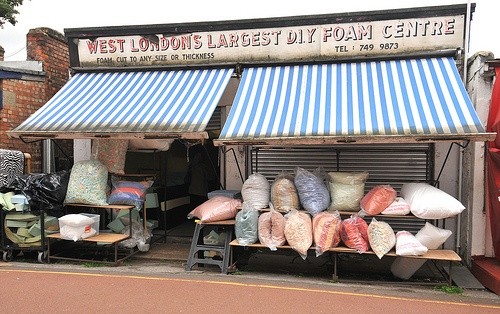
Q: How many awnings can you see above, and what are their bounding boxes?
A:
[11,67,234,139]
[212,57,497,147]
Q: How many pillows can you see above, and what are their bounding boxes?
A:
[187,167,467,260]
[109,177,154,210]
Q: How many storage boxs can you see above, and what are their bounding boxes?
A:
[58,213,100,240]
[3,192,30,211]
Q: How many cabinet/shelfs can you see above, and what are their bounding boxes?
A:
[47,199,147,264]
[228,206,462,287]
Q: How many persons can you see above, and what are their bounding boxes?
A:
[184,153,214,224]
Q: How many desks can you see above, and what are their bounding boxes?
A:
[187,220,235,274]
[0,209,48,251]
